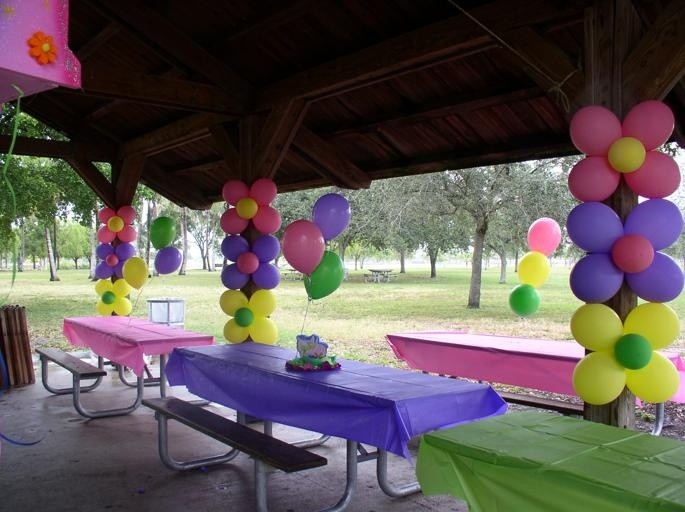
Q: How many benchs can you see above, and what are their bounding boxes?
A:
[142,393,326,512]
[494,389,588,417]
[281,266,398,283]
[34,346,103,421]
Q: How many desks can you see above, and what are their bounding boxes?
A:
[164,337,498,510]
[63,308,214,414]
[407,404,684,510]
[380,328,685,436]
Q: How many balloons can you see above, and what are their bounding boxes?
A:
[220,177,350,344]
[94,206,182,317]
